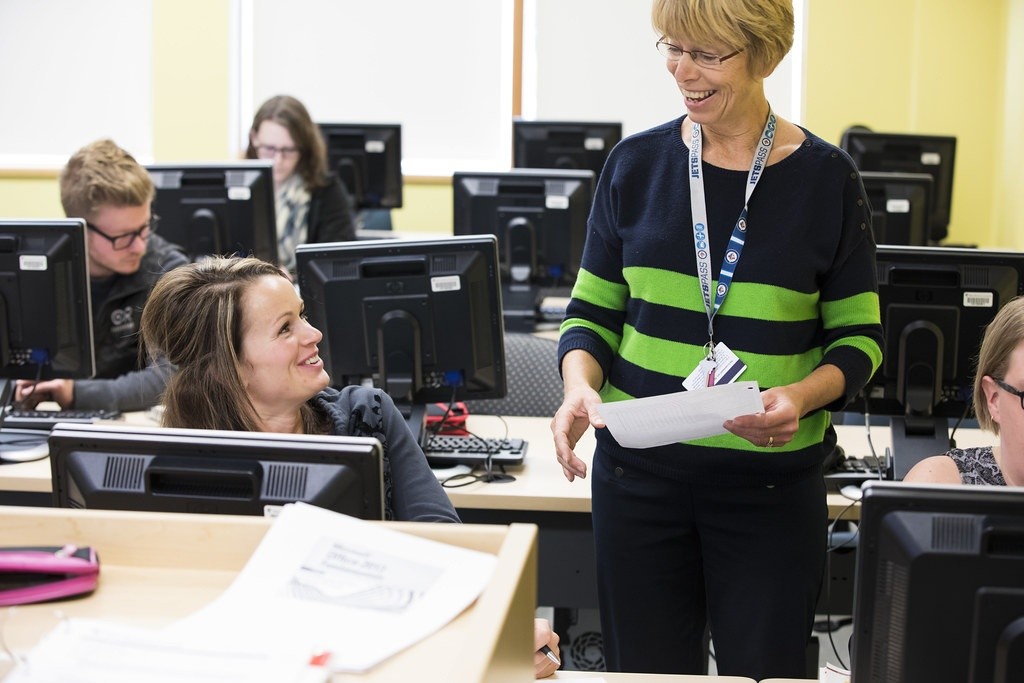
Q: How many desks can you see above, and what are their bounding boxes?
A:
[0,502,539,681]
[0,364,994,520]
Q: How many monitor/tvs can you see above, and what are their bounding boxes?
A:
[0,123,1024,683]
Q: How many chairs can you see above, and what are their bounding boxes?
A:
[451,331,566,418]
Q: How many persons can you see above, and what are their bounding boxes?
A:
[901,296,1024,490]
[243,92,359,281]
[19,137,193,414]
[139,251,560,678]
[554,1,884,680]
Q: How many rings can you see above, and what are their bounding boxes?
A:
[767,436,774,446]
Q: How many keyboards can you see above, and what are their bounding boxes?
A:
[825,454,893,489]
[425,434,529,466]
[3,408,121,429]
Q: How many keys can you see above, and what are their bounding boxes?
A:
[704,358,717,375]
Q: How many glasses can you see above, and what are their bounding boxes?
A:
[86,213,161,250]
[656,34,754,69]
[255,144,306,161]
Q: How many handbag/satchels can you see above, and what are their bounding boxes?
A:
[0,546,99,607]
